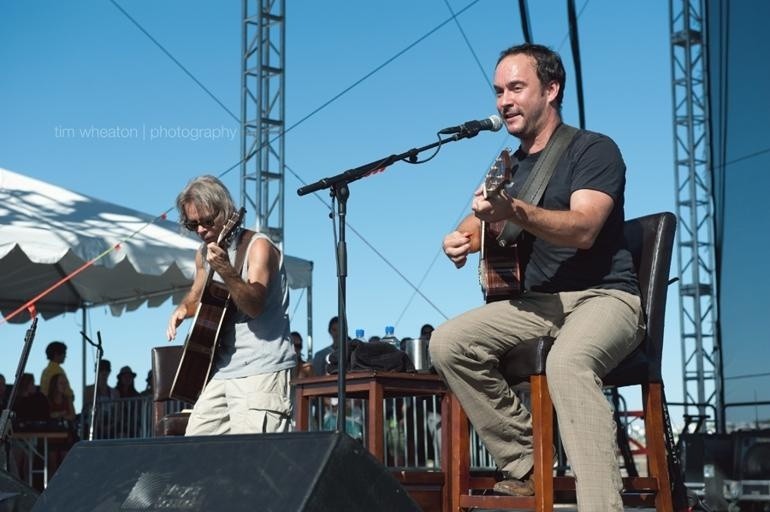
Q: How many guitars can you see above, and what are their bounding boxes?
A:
[168,208,246,404]
[476,148,520,302]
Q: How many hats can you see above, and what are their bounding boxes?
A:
[146,370,152,381]
[117,367,136,380]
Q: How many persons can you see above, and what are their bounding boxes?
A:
[141,367,154,439]
[83,359,121,441]
[166,173,300,439]
[114,365,143,438]
[15,373,49,420]
[40,340,76,420]
[0,375,11,418]
[290,314,440,467]
[10,373,38,484]
[427,41,646,512]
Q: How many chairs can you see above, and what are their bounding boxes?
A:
[450,211,678,511]
[150,344,192,436]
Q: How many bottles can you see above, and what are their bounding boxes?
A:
[379,326,401,352]
[353,329,368,343]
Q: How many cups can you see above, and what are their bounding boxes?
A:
[404,338,433,374]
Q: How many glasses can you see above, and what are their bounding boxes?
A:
[184,211,220,231]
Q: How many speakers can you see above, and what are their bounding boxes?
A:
[0,466,39,511]
[732,428,770,510]
[686,434,733,511]
[26,430,419,512]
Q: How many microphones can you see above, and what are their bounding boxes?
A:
[442,115,503,140]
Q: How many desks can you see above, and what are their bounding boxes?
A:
[9,432,71,489]
[287,368,451,512]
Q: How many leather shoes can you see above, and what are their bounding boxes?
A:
[490,443,562,498]
